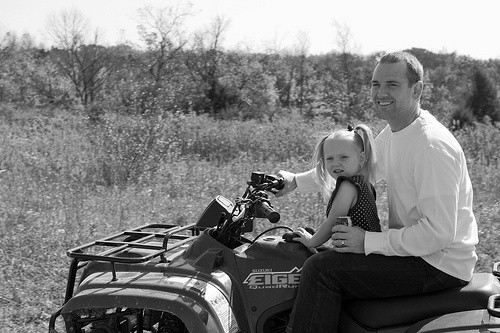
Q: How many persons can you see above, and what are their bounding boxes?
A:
[273,50,479,332]
[293,123,381,254]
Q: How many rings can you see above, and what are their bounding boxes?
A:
[343,238,345,246]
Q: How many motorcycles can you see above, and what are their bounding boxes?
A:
[48,170,500,333]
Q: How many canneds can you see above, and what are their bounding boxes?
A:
[334,217,352,247]
